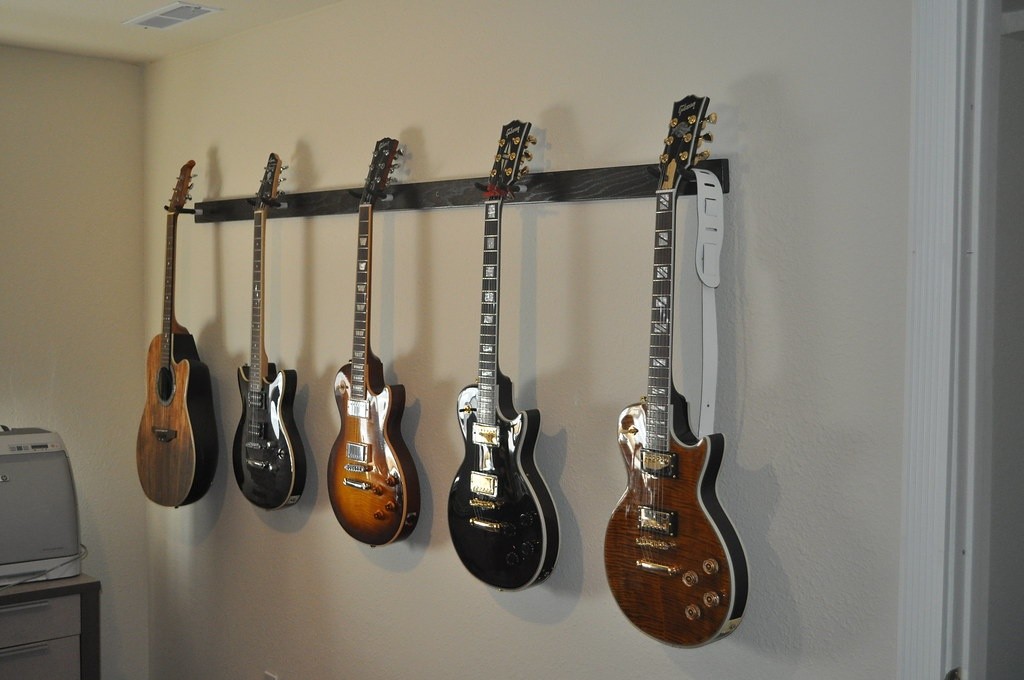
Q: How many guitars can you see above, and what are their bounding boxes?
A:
[136,160,219,507]
[600,94,753,650]
[325,136,424,546]
[230,150,308,509]
[444,119,562,591]
